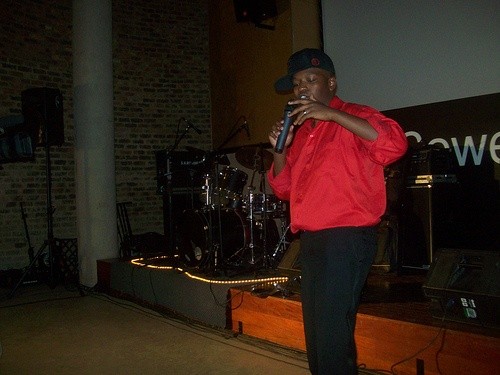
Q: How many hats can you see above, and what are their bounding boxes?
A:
[274,48,335,94]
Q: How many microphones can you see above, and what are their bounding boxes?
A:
[275,104,295,153]
[183,119,201,135]
[242,117,251,140]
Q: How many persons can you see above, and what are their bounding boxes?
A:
[266,48,408,375]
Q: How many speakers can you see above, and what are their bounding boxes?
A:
[397,183,460,269]
[162,191,204,253]
[233,0,276,23]
[22,88,65,148]
[420,248,500,330]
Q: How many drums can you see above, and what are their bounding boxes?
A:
[241,193,286,219]
[214,165,249,201]
[175,207,254,268]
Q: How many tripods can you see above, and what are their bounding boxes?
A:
[143,125,194,269]
[204,114,290,275]
[3,148,75,300]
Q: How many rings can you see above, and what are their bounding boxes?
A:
[302,110,306,115]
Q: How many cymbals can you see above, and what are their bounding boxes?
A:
[235,146,274,171]
